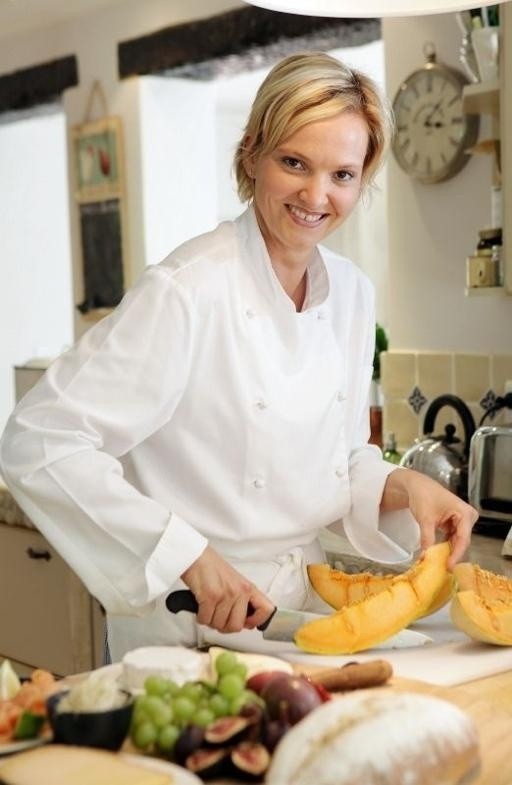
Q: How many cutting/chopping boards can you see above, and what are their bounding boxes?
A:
[184,597,512,688]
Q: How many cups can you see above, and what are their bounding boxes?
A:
[469,26,501,82]
[117,645,202,695]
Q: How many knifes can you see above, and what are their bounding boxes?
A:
[165,585,437,653]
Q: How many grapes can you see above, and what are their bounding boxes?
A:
[130,651,265,755]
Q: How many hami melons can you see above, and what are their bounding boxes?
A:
[294,541,512,654]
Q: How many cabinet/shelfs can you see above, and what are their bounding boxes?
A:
[0,522,92,678]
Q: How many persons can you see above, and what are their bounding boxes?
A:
[1,49,483,679]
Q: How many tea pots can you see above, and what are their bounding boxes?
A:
[394,393,476,505]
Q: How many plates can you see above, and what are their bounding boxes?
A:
[93,649,296,714]
[0,720,58,760]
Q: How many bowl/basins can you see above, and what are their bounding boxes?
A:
[46,680,133,748]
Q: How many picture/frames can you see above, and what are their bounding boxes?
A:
[72,113,131,321]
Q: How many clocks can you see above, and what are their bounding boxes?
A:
[388,62,480,186]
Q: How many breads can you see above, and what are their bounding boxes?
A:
[265,687,482,785]
